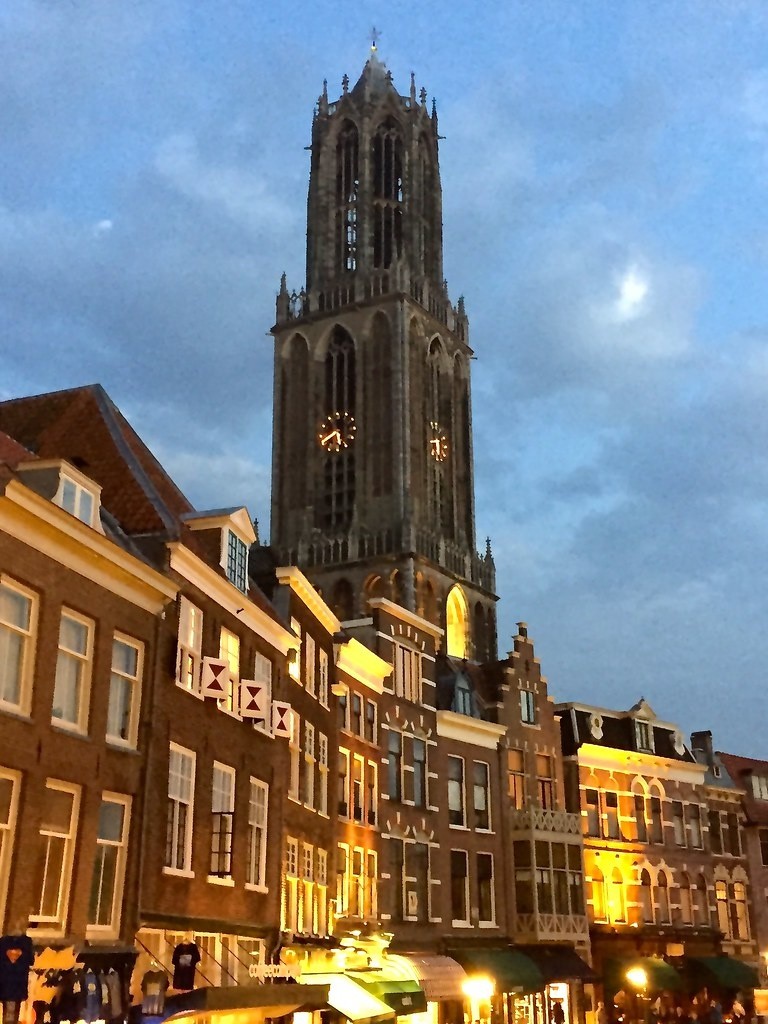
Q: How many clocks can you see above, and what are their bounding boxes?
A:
[430,422,448,459]
[319,413,357,452]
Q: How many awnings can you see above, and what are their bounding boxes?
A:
[518,944,596,981]
[603,956,689,991]
[292,973,396,1024]
[166,983,331,1013]
[345,972,428,1016]
[447,949,546,992]
[387,954,469,1001]
[687,957,761,992]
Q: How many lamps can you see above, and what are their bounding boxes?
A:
[286,648,297,663]
